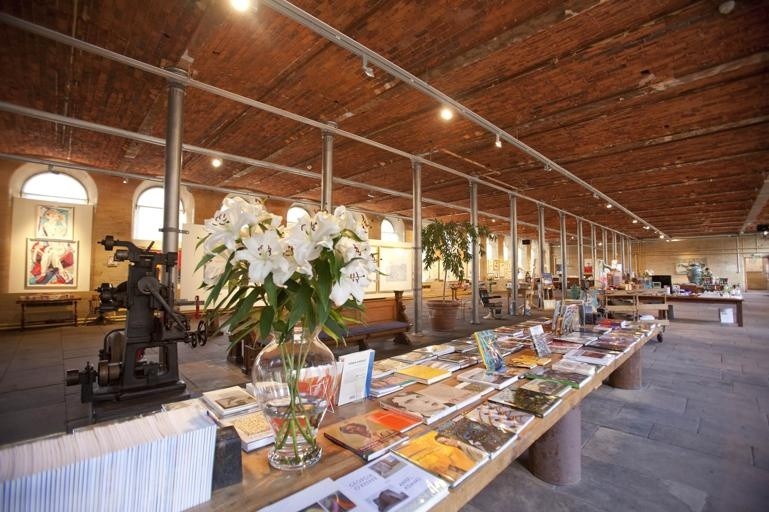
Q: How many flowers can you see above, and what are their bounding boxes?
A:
[189,197,384,458]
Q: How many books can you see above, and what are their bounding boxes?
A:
[327,345,598,488]
[2,386,447,512]
[449,302,657,368]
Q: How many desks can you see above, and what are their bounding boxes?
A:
[0,310,674,512]
[15,296,83,332]
[600,290,745,327]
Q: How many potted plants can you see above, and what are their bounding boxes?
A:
[419,219,495,331]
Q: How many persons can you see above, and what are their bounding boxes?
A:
[704,268,714,275]
[584,263,592,272]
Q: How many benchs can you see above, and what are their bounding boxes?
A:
[227,291,414,371]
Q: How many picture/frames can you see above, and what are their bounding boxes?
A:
[24,237,81,290]
[36,204,75,238]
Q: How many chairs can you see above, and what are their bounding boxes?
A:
[480,289,503,320]
